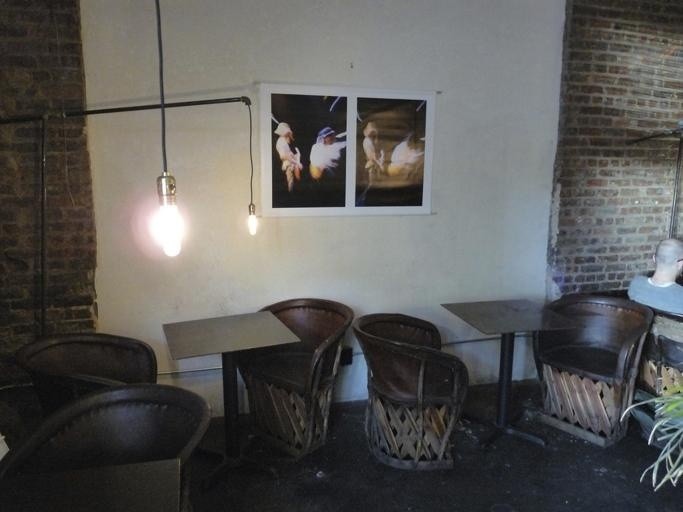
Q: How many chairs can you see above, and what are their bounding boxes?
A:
[14,332,158,423]
[241,298,356,474]
[533,292,654,447]
[351,311,472,472]
[0,384,213,511]
[657,334,683,374]
[635,306,683,451]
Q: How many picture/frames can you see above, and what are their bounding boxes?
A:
[259,83,434,217]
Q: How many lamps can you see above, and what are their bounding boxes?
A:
[153,1,186,258]
[33,97,261,333]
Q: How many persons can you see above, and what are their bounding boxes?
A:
[274,119,425,197]
[627,238,683,315]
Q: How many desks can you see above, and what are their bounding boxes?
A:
[440,298,578,451]
[160,309,295,489]
